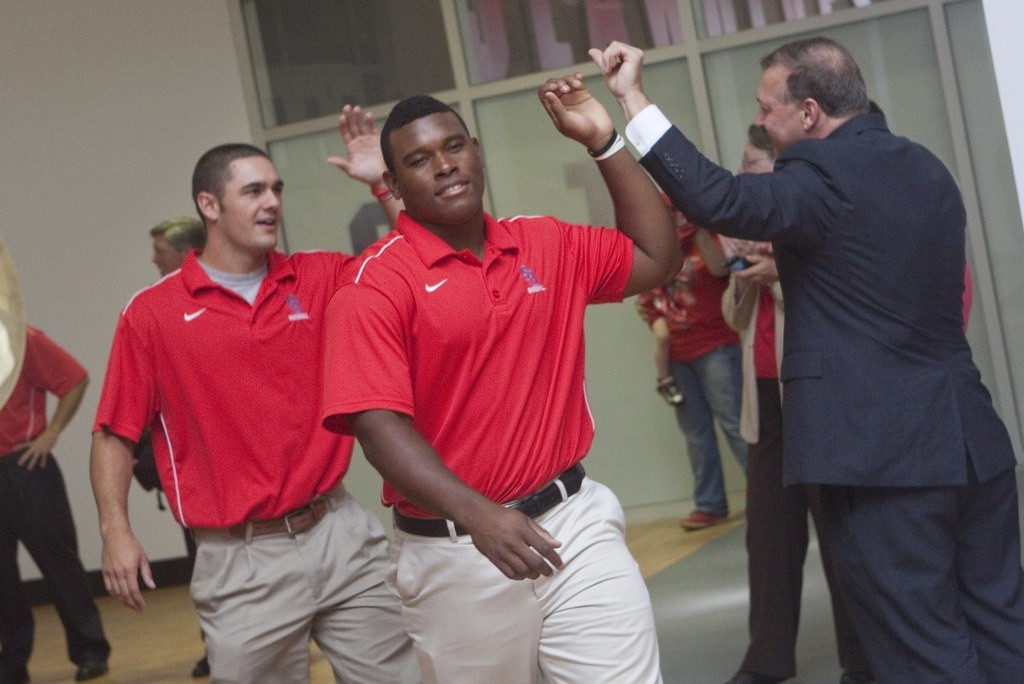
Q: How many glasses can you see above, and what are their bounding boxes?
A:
[741,156,773,169]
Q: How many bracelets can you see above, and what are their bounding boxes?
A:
[372,184,398,202]
[588,128,627,161]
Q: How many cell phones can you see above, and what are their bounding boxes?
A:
[723,256,751,272]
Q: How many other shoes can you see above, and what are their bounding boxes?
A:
[191,656,209,677]
[75,663,107,679]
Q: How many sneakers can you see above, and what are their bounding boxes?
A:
[679,510,720,530]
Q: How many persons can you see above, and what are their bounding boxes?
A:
[0,240,112,684]
[87,104,423,684]
[118,211,209,677]
[318,73,684,684]
[588,35,1023,684]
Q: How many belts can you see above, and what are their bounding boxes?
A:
[194,483,346,542]
[393,461,586,538]
